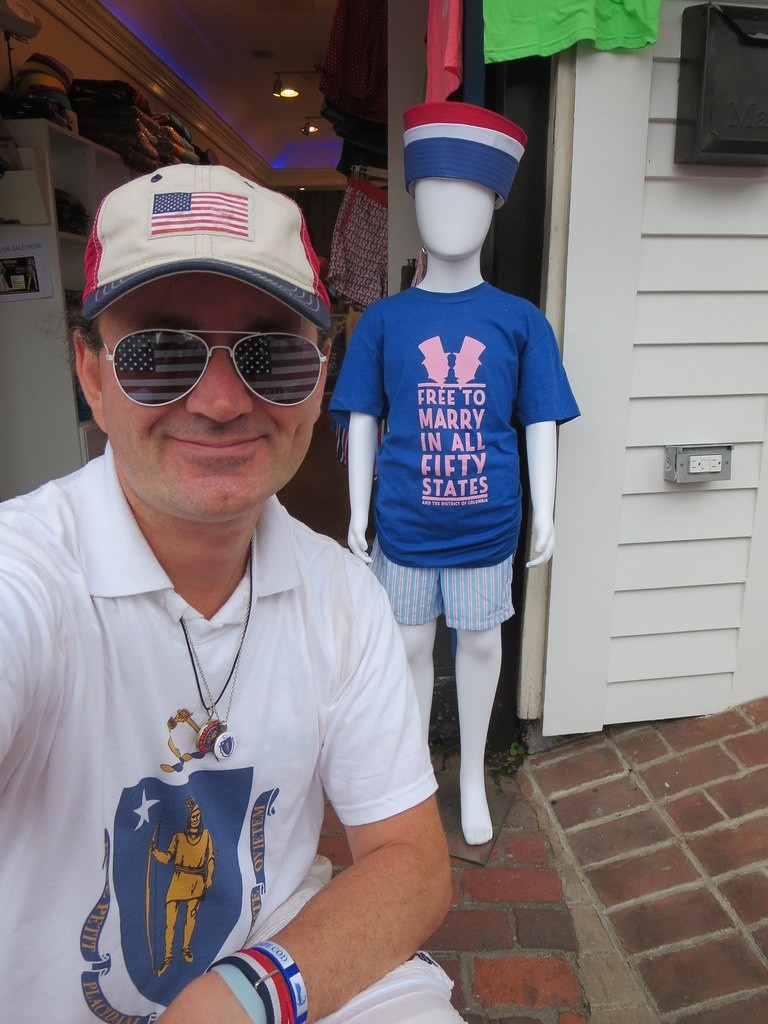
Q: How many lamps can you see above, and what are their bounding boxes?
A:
[273,73,282,98]
[301,119,310,137]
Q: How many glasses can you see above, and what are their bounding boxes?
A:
[94,327,327,407]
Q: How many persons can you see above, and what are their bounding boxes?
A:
[328,103,581,847]
[0,163,468,1024]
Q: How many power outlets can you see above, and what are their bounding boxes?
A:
[689,455,722,473]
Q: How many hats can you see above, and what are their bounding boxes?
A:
[80,162,333,335]
[400,103,529,211]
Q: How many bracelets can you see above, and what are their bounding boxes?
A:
[205,940,308,1024]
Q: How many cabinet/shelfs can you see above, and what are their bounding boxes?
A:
[0,118,130,512]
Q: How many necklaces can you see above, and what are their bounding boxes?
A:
[178,534,255,759]
